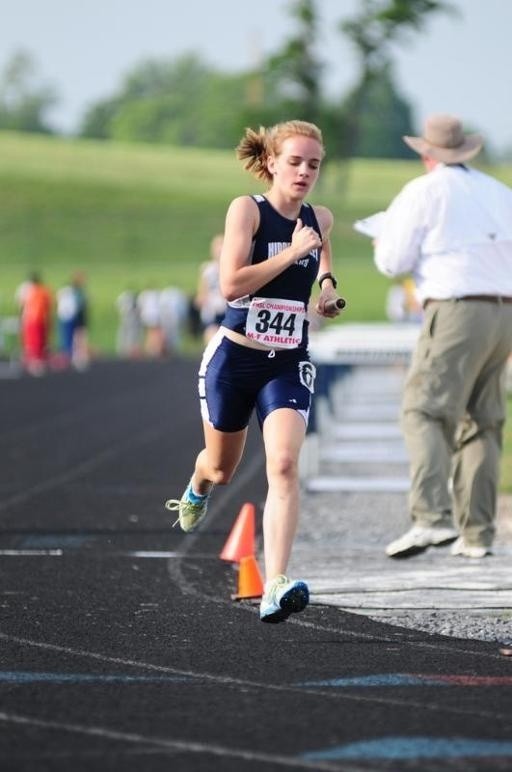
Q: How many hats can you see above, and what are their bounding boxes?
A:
[403,113,484,164]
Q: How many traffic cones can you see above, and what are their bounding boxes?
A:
[215,503,258,567]
[232,558,265,603]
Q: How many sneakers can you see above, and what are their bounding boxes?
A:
[451,538,494,557]
[385,522,460,557]
[260,576,309,624]
[166,482,209,532]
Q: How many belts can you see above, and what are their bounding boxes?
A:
[463,295,512,304]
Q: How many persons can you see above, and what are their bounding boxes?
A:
[165,119,343,625]
[366,110,512,561]
[14,235,226,380]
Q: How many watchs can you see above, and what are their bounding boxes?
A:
[318,271,337,290]
